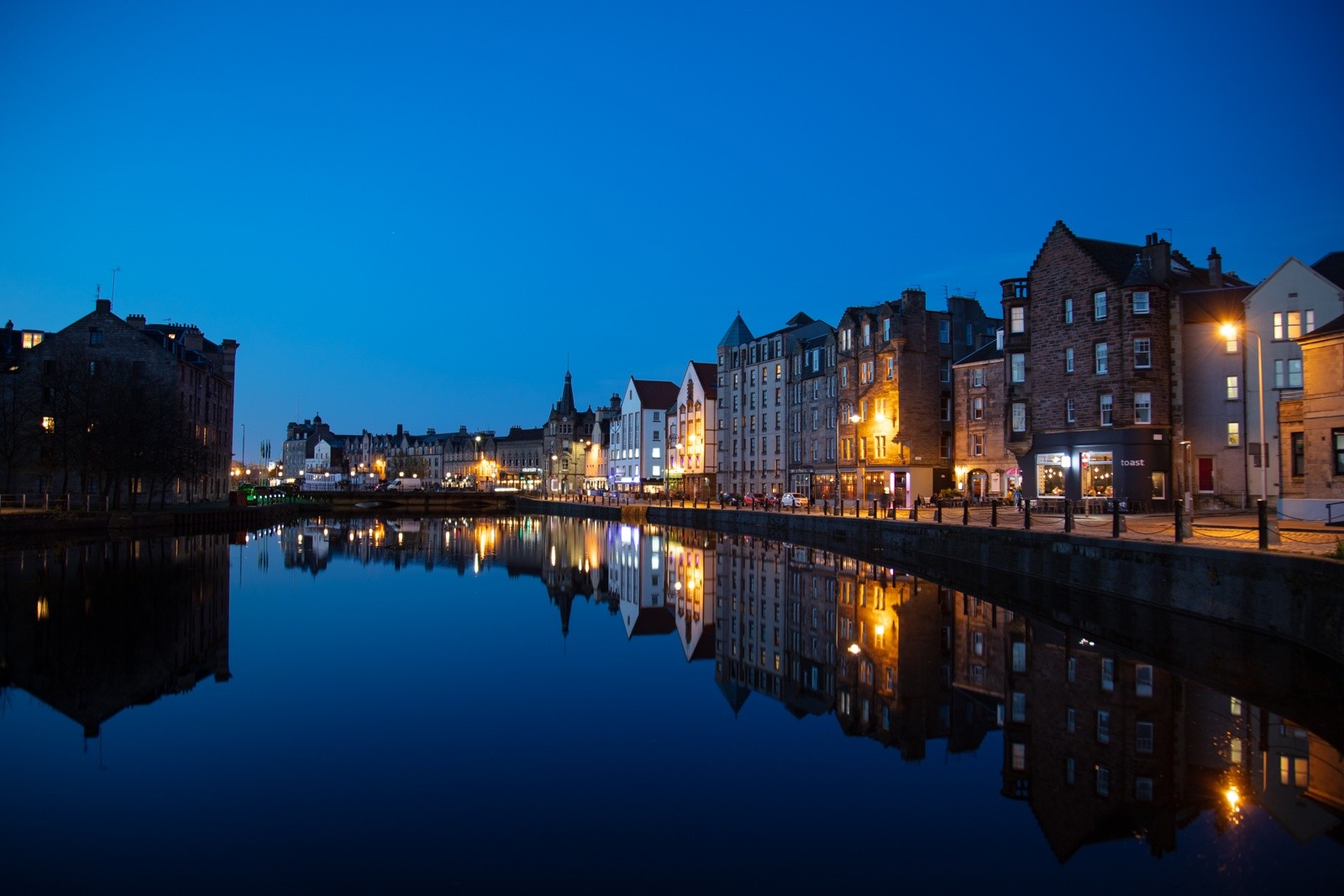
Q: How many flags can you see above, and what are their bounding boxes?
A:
[260,439,270,458]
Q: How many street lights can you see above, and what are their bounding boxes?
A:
[241,424,245,468]
[1217,322,1273,512]
[665,433,683,507]
[833,397,860,516]
[544,453,558,499]
[474,436,481,473]
[574,437,591,499]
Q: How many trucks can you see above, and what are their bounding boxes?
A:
[387,478,420,491]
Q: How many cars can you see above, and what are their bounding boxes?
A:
[765,493,783,507]
[250,486,282,498]
[273,482,300,491]
[236,483,260,495]
[375,483,388,492]
[743,493,766,507]
[723,493,744,507]
[780,493,809,507]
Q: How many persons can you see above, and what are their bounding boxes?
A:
[823,499,829,515]
[1087,483,1113,497]
[930,491,941,507]
[763,499,768,512]
[719,491,724,504]
[878,489,887,518]
[776,492,784,498]
[1006,486,1021,511]
[607,487,611,496]
[334,482,351,491]
[1052,485,1065,496]
[577,486,583,502]
[810,495,814,506]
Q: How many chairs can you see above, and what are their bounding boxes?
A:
[1037,499,1103,514]
[924,497,936,508]
